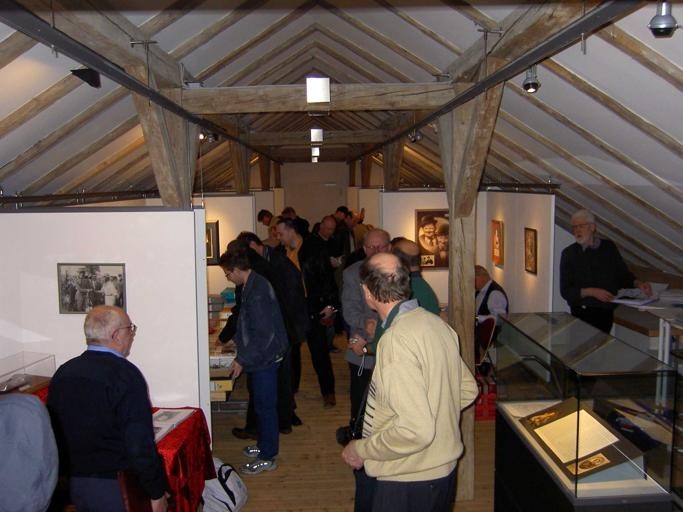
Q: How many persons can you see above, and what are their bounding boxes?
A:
[46,305,171,511]
[341,253,479,512]
[559,209,653,327]
[215,205,442,474]
[474,265,509,374]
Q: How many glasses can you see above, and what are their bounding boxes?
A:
[112,323,136,340]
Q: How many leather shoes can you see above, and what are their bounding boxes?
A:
[232,428,257,440]
[279,410,302,434]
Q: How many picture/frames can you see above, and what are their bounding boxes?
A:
[525,228,537,275]
[57,262,126,314]
[206,219,220,265]
[491,219,504,267]
[414,208,449,270]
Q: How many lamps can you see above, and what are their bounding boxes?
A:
[70,68,102,90]
[646,0,678,40]
[522,62,541,94]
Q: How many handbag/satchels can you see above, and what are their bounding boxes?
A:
[202,457,248,512]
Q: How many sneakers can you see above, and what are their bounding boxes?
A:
[323,393,336,407]
[239,459,277,475]
[242,445,260,458]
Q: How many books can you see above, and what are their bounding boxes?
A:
[152,409,196,445]
[609,280,683,331]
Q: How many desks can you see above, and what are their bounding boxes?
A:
[36,387,218,512]
